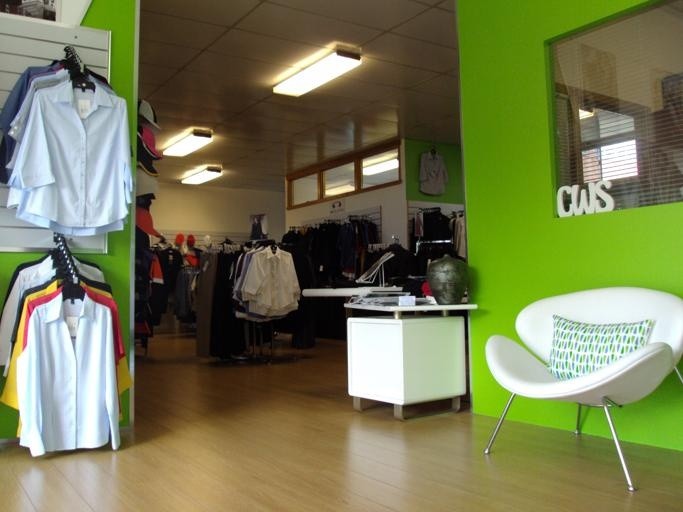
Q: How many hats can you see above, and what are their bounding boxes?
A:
[204,235,212,248]
[175,234,184,245]
[187,235,195,248]
[135,97,162,239]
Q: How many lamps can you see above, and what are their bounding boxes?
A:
[163,129,223,185]
[362,157,400,175]
[324,181,356,198]
[272,50,363,98]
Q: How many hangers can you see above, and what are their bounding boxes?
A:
[50,236,85,303]
[60,45,95,93]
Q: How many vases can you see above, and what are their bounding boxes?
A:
[427,254,470,305]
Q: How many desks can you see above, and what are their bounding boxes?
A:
[342,302,478,421]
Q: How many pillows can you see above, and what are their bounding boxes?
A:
[546,314,655,383]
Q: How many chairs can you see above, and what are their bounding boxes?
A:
[485,286,683,491]
